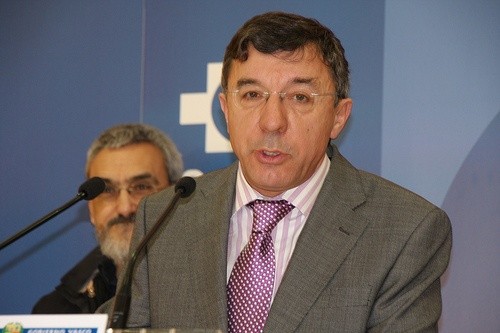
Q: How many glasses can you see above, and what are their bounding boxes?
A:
[96,183,166,199]
[227,85,345,109]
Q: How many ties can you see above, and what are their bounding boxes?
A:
[226,199,296,332]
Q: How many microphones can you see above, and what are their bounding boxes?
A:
[0,177,106,250]
[110,176,196,330]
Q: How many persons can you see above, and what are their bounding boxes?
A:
[105,11,453,333]
[32,125,183,314]
